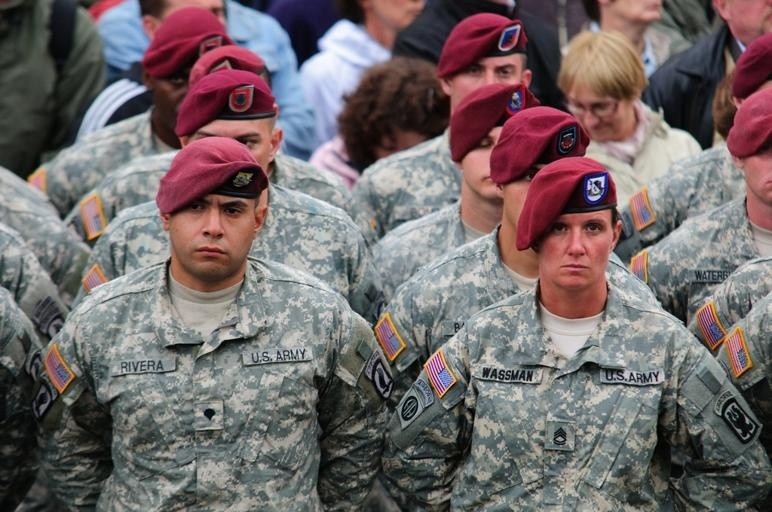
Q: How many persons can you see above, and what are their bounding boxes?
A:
[0,0,771,383]
[377,156,771,512]
[33,137,393,511]
[0,283,42,511]
[712,289,772,463]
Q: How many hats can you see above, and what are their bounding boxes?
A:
[732,32,772,98]
[436,12,527,77]
[727,89,772,158]
[490,105,590,184]
[156,137,268,213]
[450,83,539,161]
[188,45,265,87]
[515,160,617,251]
[143,7,233,79]
[175,69,276,136]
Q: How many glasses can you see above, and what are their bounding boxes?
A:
[560,94,621,116]
